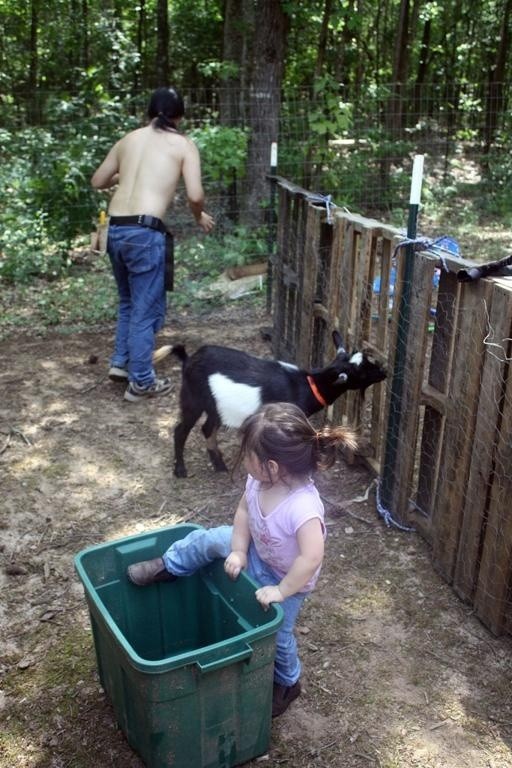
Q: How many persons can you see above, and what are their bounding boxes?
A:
[90,87,217,403]
[126,400,345,717]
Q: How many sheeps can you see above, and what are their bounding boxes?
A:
[150,330,389,479]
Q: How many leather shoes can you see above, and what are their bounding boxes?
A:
[127,557,176,587]
[272,680,300,718]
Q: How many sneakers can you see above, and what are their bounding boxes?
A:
[108,368,128,382]
[124,376,174,402]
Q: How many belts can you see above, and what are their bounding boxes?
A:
[109,216,173,291]
